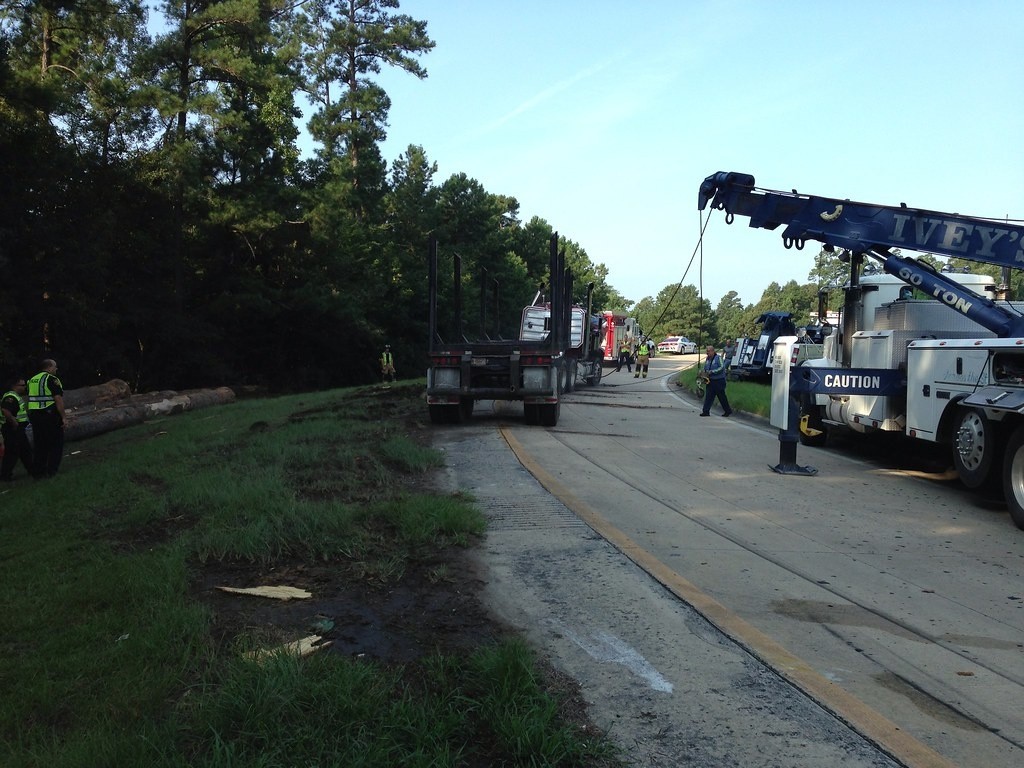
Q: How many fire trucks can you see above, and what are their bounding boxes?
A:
[597,310,643,364]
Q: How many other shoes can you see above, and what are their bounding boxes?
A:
[700,412,710,417]
[722,408,732,417]
[0,473,17,481]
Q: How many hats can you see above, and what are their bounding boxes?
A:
[640,337,646,342]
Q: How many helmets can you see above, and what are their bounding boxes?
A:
[384,344,392,349]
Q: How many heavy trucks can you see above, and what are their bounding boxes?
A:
[424,230,610,426]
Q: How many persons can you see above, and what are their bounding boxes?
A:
[26,359,68,479]
[616,334,632,372]
[634,337,650,378]
[0,377,32,482]
[701,346,732,419]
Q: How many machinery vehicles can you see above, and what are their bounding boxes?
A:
[696,171,1024,529]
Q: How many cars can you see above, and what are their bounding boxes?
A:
[658,334,698,355]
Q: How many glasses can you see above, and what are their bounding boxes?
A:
[17,384,26,386]
